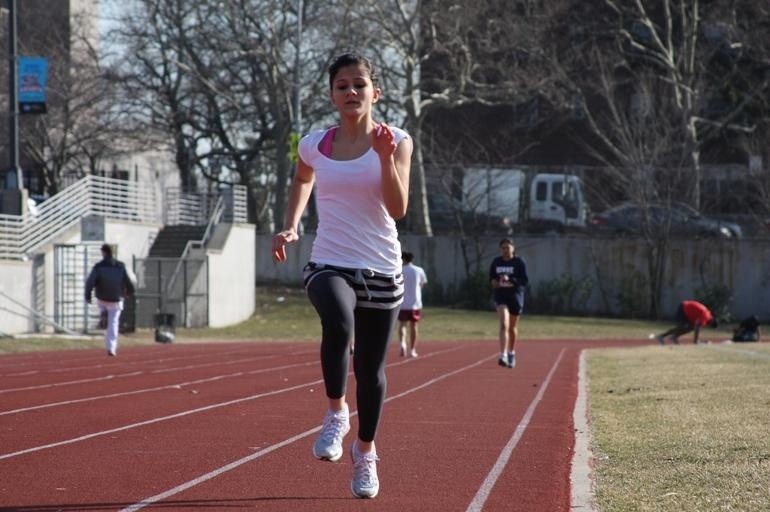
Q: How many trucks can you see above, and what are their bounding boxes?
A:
[461,168,590,238]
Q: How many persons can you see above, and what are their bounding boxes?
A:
[268,52,416,502]
[80,242,136,357]
[397,251,429,359]
[487,237,530,369]
[655,299,718,346]
[731,313,762,343]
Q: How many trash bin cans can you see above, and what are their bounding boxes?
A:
[100,266,136,334]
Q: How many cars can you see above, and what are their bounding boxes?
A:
[585,200,742,239]
[397,192,513,238]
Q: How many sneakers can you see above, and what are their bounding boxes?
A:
[313,402,350,462]
[498,353,516,368]
[656,333,680,345]
[400,342,419,357]
[350,440,379,499]
